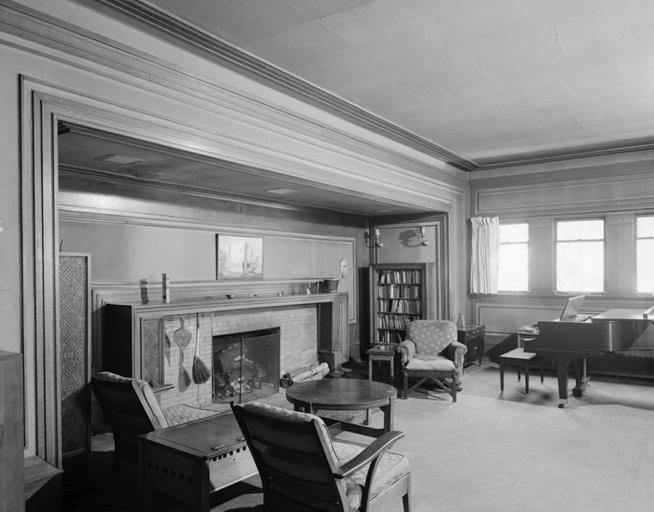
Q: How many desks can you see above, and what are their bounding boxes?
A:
[136,411,258,512]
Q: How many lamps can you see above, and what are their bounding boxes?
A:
[420,226,429,246]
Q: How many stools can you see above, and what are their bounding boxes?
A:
[401,359,458,402]
[498,348,544,394]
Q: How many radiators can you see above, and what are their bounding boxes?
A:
[589,349,653,379]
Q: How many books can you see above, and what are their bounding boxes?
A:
[376,267,421,344]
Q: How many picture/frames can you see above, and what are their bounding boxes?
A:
[216,234,264,281]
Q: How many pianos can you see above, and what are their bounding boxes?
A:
[523,295,654,408]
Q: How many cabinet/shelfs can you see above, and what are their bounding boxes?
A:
[368,263,427,347]
[457,323,488,367]
[517,327,539,347]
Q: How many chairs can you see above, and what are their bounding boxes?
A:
[397,320,468,392]
[91,372,224,473]
[230,399,411,512]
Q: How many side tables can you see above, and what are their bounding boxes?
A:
[366,344,398,381]
